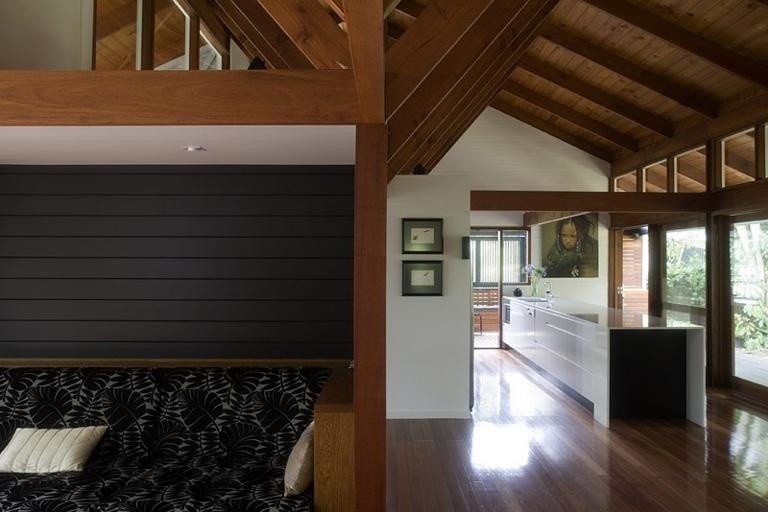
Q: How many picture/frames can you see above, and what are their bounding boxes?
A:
[401,260,443,295]
[401,217,443,254]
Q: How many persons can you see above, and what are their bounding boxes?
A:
[545,215,598,277]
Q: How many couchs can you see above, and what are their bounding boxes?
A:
[0,358,355,512]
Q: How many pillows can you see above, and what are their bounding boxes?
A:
[281,419,314,497]
[0,424,108,474]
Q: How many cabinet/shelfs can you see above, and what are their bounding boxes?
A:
[502,295,708,430]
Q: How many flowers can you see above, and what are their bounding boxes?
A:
[526,264,547,276]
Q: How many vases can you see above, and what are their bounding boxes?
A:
[530,270,542,297]
